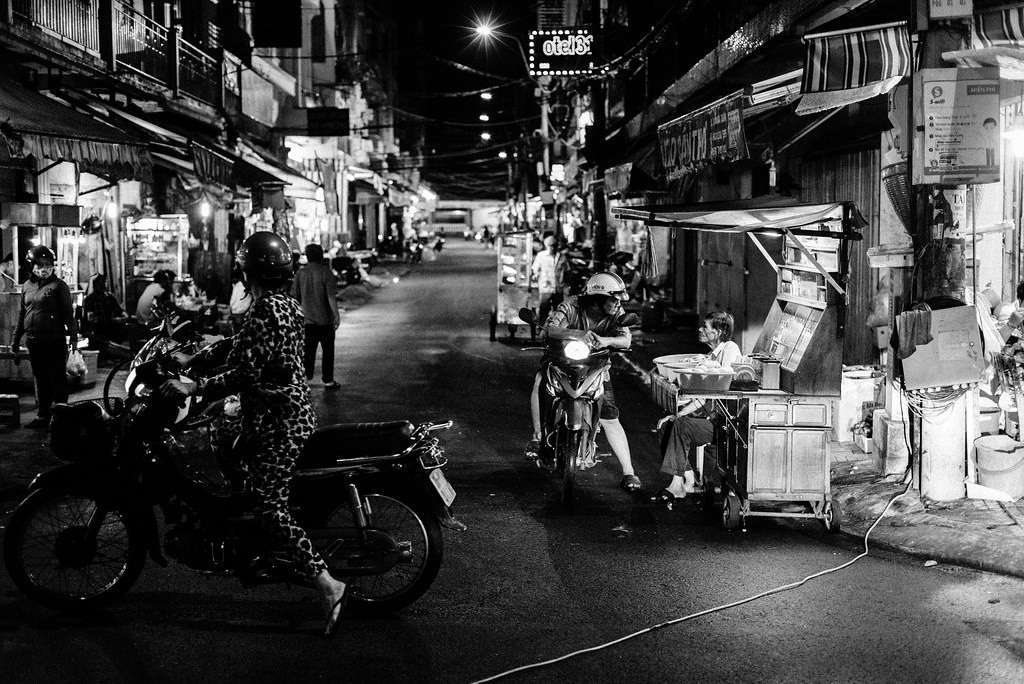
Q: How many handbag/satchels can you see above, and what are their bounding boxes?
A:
[65,348,90,380]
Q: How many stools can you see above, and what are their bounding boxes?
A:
[0,394,20,429]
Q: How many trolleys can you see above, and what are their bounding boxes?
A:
[648,373,842,534]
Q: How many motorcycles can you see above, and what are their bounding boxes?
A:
[1,311,469,616]
[517,308,638,505]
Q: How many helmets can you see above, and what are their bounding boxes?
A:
[584,272,630,301]
[233,231,294,291]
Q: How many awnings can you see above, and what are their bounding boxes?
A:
[610,201,844,299]
[660,69,804,180]
[0,38,328,205]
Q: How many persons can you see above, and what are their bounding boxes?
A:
[290,244,341,389]
[157,230,351,639]
[522,270,643,491]
[529,235,570,326]
[8,240,178,425]
[650,310,742,503]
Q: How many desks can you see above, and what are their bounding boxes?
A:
[0,345,39,405]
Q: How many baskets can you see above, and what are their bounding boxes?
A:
[52,396,123,461]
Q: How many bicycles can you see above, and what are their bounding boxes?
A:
[103,354,200,428]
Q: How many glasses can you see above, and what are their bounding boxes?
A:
[35,261,50,268]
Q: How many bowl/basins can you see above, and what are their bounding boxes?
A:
[662,363,695,382]
[673,369,736,391]
[652,354,711,376]
[632,504,672,523]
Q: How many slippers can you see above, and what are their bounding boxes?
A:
[648,489,683,504]
[524,439,542,461]
[320,583,351,637]
[621,475,641,493]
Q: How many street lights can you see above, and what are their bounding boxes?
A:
[477,24,550,179]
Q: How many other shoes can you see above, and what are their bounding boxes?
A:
[324,380,340,393]
[24,418,50,429]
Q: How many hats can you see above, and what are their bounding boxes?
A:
[544,235,557,245]
[24,245,55,266]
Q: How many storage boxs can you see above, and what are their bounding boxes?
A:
[78,350,100,384]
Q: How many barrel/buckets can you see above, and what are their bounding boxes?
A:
[979,407,1002,436]
[970,435,1023,499]
